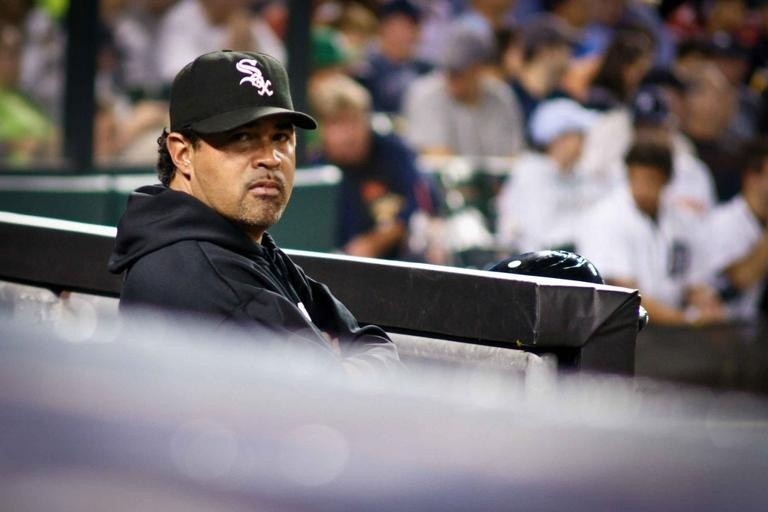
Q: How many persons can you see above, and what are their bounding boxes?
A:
[107,49,410,380]
[1,1,768,327]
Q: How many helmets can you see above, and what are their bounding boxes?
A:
[484,249,648,332]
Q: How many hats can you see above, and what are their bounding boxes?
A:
[528,96,599,145]
[169,49,317,134]
[524,26,594,56]
